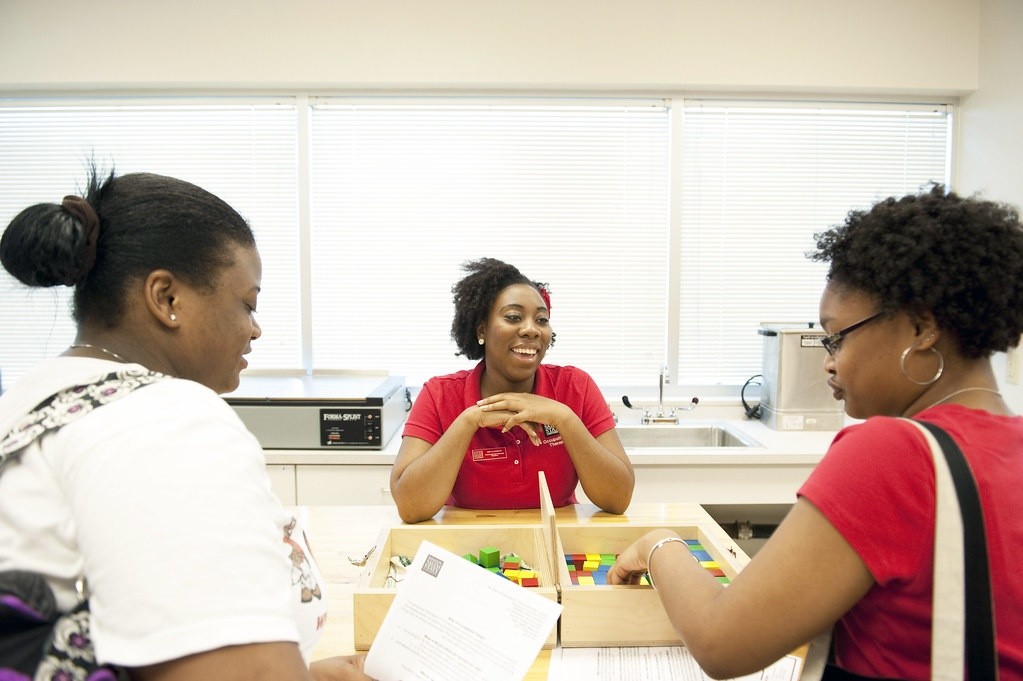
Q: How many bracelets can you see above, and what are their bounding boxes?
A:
[647,537,689,591]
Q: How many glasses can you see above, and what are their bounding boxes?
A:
[821,304,894,358]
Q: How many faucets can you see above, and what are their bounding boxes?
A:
[621,361,700,426]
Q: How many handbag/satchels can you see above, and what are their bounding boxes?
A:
[0,568,119,681]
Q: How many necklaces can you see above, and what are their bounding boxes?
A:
[69,344,128,363]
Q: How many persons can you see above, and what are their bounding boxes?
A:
[0,172,373,681]
[389,258,635,523]
[606,186,1023,681]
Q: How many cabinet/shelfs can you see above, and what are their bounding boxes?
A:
[266,464,396,505]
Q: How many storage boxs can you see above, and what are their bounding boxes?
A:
[353,524,558,651]
[556,521,737,648]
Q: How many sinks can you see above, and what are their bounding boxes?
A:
[614,418,769,451]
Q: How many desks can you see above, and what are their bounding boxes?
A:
[274,501,811,681]
